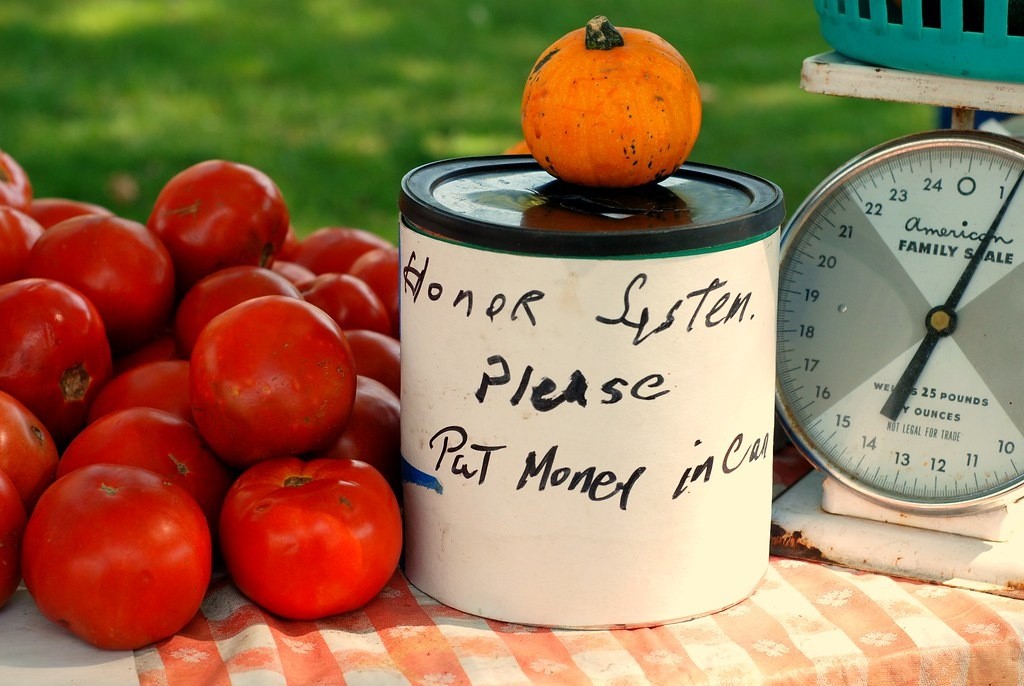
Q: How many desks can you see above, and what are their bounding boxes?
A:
[1,412,1018,685]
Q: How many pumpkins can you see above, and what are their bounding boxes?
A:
[517,15,704,190]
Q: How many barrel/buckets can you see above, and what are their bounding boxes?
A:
[389,157,786,630]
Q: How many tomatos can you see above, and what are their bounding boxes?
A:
[0,147,402,651]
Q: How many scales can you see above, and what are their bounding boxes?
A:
[770,43,1023,602]
